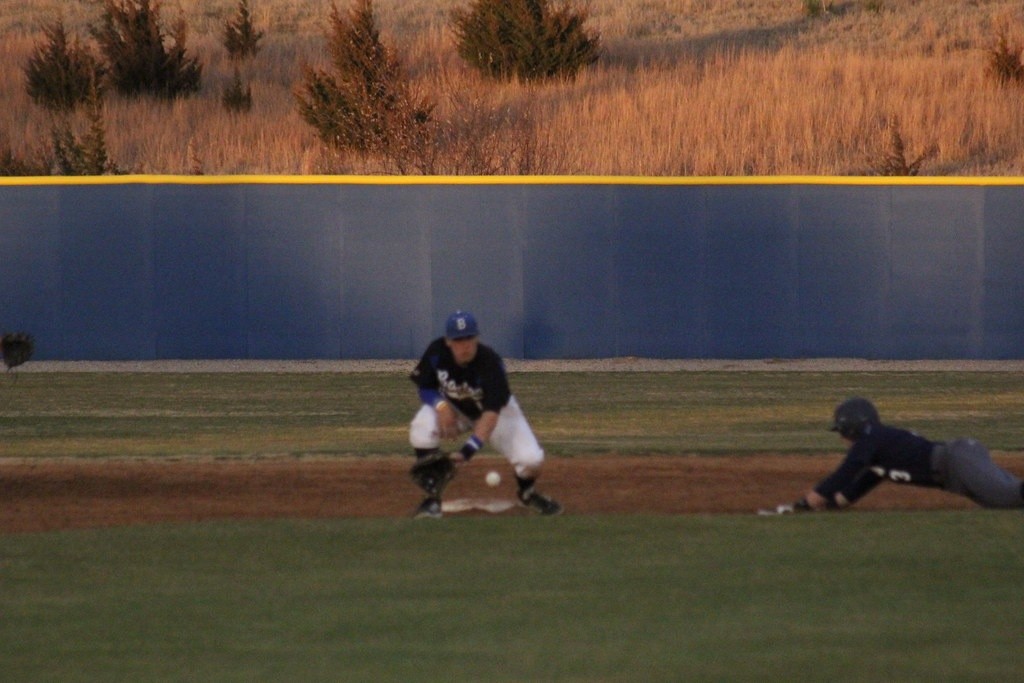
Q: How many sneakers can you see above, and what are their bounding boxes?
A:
[517,488,563,516]
[414,499,442,520]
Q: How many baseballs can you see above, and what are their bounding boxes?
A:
[485,471,501,488]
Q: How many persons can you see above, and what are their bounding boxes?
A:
[777,398,1024,511]
[408,311,561,518]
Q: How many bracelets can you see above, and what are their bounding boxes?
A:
[435,401,446,410]
[460,437,482,460]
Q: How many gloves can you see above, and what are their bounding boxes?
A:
[780,497,811,512]
[816,498,842,512]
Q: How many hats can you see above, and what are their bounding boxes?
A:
[445,310,479,340]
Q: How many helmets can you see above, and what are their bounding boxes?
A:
[831,397,879,442]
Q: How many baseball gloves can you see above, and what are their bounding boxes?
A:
[0,332,34,368]
[408,452,458,500]
